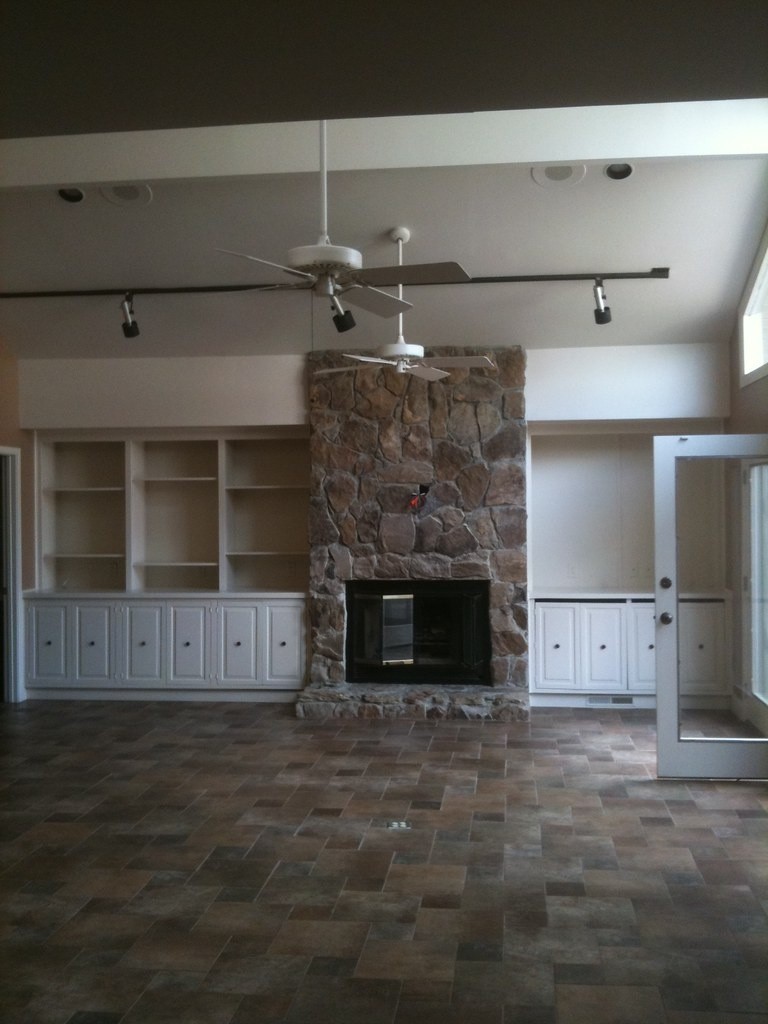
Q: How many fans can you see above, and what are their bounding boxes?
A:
[315,226,495,384]
[196,119,468,320]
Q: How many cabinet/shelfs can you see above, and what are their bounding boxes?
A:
[19,442,308,701]
[531,598,733,714]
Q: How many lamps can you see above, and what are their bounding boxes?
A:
[329,295,355,332]
[120,297,140,336]
[592,281,611,324]
